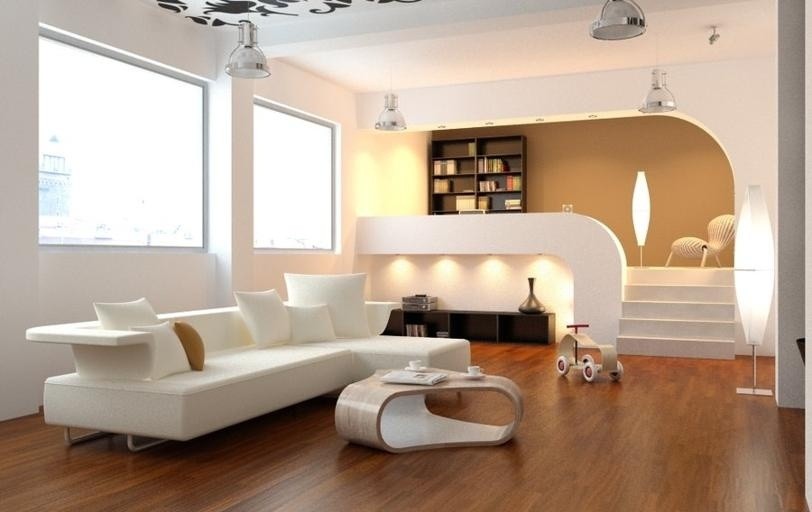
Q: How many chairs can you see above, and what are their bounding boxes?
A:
[660,210,735,272]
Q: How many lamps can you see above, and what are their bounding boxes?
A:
[372,93,409,131]
[637,69,678,114]
[224,21,271,79]
[729,183,779,399]
[630,168,655,266]
[589,1,647,41]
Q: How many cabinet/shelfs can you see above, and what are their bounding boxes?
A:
[431,134,528,215]
[379,311,556,348]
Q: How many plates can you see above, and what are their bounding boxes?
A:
[462,373,485,379]
[405,367,429,371]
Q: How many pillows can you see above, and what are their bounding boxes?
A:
[172,317,208,373]
[232,284,294,350]
[283,271,368,340]
[127,319,194,382]
[285,300,337,344]
[90,295,161,331]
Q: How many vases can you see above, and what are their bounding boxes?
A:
[516,275,548,315]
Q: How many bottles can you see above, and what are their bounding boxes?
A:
[517,277,546,314]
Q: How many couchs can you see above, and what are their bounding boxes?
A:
[22,298,477,455]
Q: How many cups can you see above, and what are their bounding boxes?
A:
[468,364,480,376]
[409,361,420,367]
[560,204,574,214]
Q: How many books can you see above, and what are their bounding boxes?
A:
[380,370,447,386]
[478,156,522,209]
[432,142,475,211]
